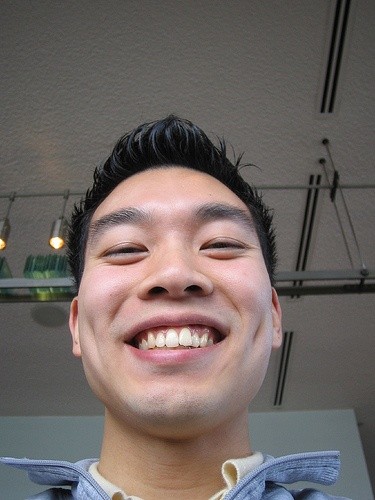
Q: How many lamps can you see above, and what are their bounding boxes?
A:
[48,197,70,250]
[0,197,14,250]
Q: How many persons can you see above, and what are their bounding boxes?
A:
[0,111,341,499]
